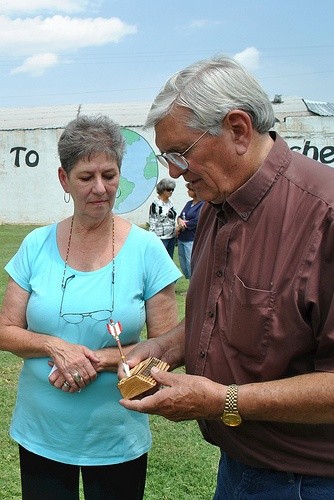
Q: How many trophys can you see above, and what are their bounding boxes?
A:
[107,319,171,400]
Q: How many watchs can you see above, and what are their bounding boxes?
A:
[220,384,242,427]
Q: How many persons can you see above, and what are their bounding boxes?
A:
[117,57,334,500]
[0,114,184,500]
[148,179,176,263]
[177,182,206,281]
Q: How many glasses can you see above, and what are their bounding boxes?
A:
[155,122,216,171]
[164,188,174,192]
[59,273,114,324]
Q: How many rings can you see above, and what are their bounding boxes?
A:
[72,373,82,384]
[63,382,71,388]
